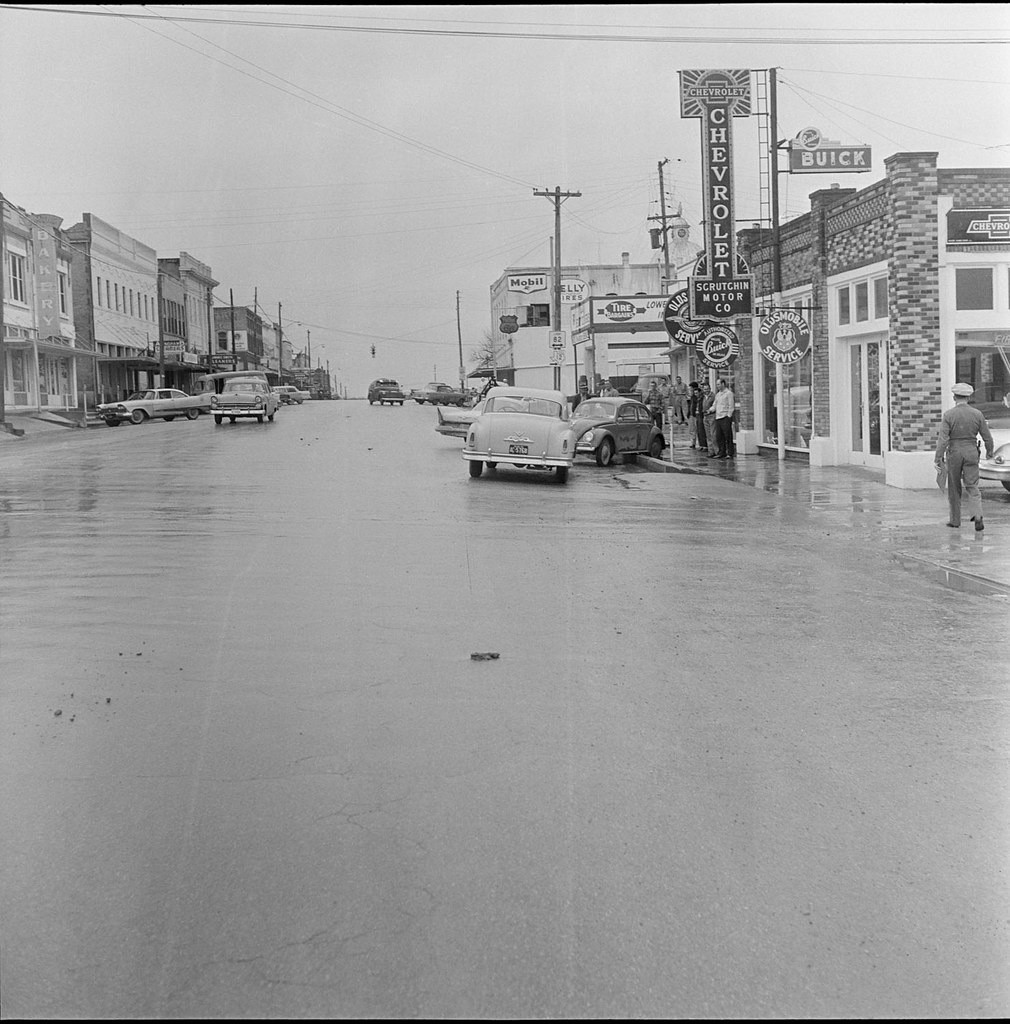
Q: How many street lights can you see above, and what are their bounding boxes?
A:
[277,320,302,386]
[309,345,326,368]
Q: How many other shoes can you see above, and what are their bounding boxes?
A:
[713,454,724,459]
[689,444,695,448]
[974,515,984,531]
[947,523,959,528]
[725,455,733,459]
[697,446,702,451]
[707,454,715,458]
[701,447,708,452]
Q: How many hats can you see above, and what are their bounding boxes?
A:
[689,382,698,387]
[951,382,974,397]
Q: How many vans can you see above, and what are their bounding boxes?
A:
[190,370,269,413]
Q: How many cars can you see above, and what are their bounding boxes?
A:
[271,384,311,407]
[368,377,406,406]
[208,376,277,424]
[410,381,483,408]
[461,386,576,484]
[977,427,1010,492]
[572,396,666,468]
[94,388,211,427]
[967,383,1010,420]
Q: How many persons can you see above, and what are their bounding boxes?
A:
[571,378,619,414]
[643,375,735,461]
[933,382,995,531]
